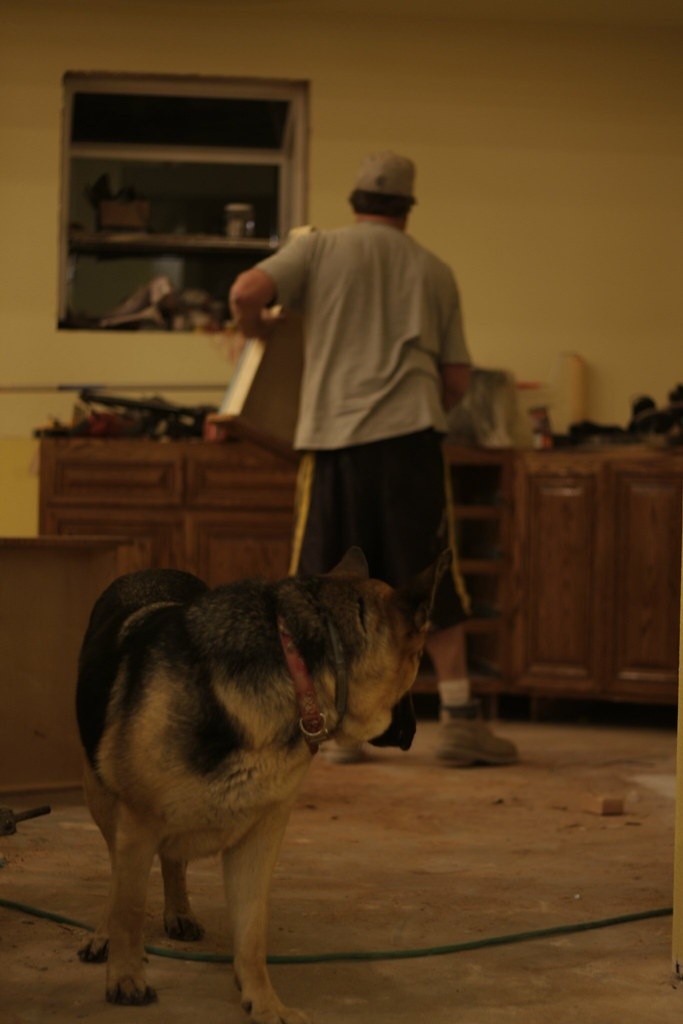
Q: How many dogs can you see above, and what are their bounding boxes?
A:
[74,546,452,1024]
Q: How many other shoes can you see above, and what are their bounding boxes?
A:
[321,741,366,761]
[429,709,521,768]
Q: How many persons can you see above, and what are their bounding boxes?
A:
[230,151,519,766]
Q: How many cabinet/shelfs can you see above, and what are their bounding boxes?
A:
[56,70,311,333]
[39,436,683,707]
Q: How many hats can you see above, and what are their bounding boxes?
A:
[349,149,420,205]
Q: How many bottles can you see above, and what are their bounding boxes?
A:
[224,203,256,237]
